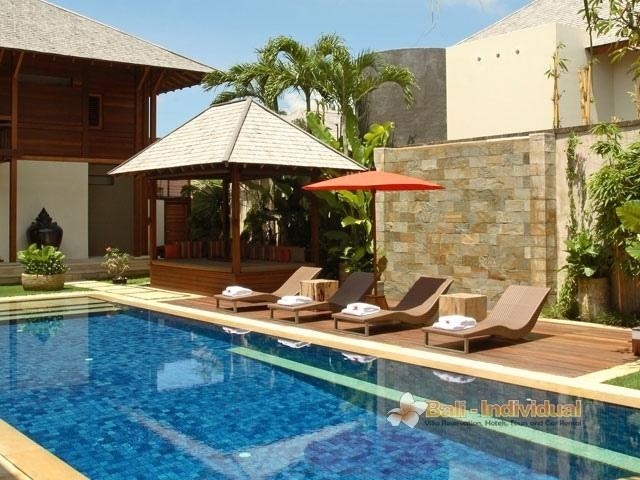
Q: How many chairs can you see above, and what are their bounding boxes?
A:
[213,265,552,355]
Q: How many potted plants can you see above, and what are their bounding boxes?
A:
[552,224,612,322]
[16,243,70,290]
[101,246,131,285]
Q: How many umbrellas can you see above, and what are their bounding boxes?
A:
[300,359,436,479]
[304,165,447,306]
